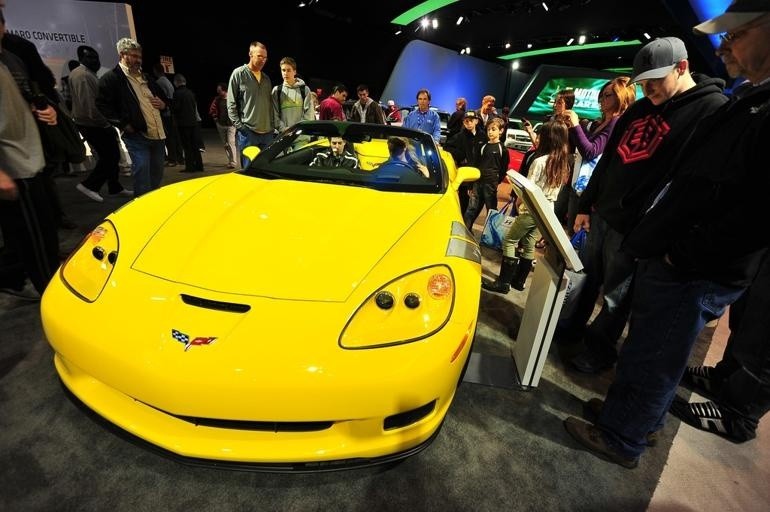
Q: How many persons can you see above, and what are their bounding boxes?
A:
[1,12,204,296]
[484,0,770,472]
[211,42,510,235]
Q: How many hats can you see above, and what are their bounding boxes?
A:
[626,37,689,85]
[693,0,770,35]
[464,111,477,118]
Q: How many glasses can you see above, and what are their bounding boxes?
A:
[721,21,770,41]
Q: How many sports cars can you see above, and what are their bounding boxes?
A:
[36,116,485,474]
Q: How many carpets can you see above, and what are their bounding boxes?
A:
[0,172,716,510]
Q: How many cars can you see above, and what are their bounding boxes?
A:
[311,101,544,153]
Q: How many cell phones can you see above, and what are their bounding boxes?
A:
[521,117,530,126]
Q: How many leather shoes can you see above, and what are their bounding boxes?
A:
[565,399,657,467]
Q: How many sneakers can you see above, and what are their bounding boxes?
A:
[680,365,747,444]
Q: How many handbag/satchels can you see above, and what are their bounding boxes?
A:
[560,229,588,320]
[480,196,520,250]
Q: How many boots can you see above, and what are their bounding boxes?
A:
[481,256,532,293]
